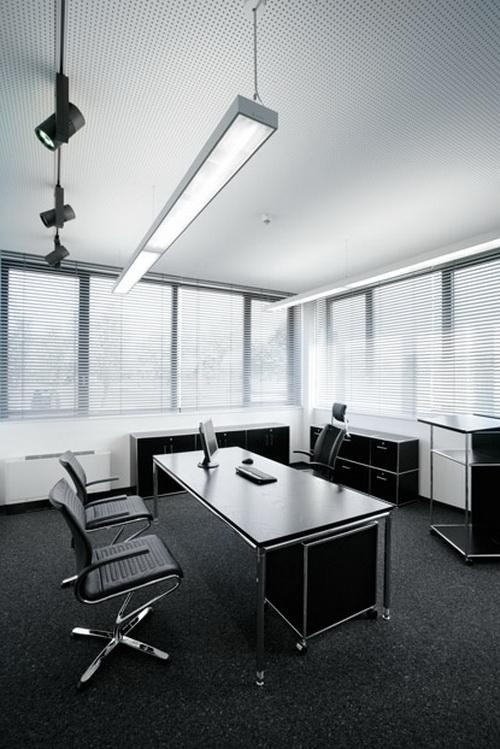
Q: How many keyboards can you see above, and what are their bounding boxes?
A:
[238,466,280,484]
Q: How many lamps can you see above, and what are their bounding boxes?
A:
[40,185,76,230]
[44,236,69,269]
[110,9,278,295]
[34,72,85,152]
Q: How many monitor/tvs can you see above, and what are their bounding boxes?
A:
[197,417,221,468]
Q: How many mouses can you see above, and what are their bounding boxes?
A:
[242,458,254,463]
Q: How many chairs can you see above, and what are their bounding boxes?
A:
[286,403,348,483]
[48,477,185,691]
[58,449,154,548]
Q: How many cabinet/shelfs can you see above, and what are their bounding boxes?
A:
[129,431,196,501]
[308,425,420,507]
[197,425,247,451]
[417,414,500,566]
[247,425,289,467]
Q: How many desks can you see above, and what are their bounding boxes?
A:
[153,445,397,686]
